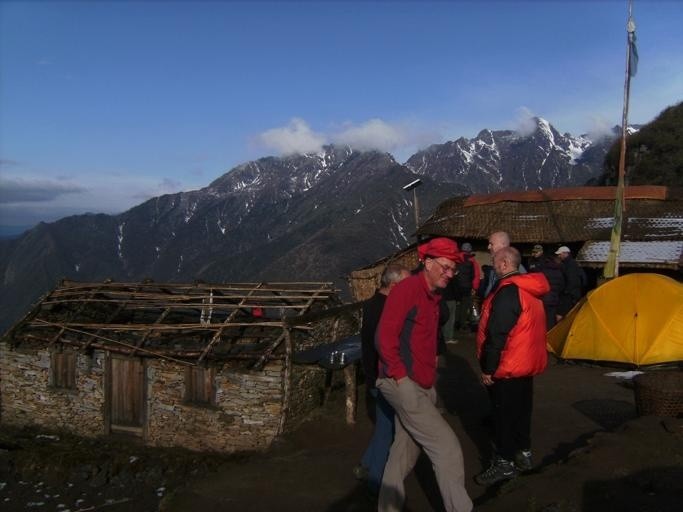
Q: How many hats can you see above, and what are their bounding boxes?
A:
[417,238,464,264]
[461,243,472,252]
[531,245,543,253]
[555,246,571,254]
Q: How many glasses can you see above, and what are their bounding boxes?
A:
[434,259,460,276]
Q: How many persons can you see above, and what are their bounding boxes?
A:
[462,232,549,486]
[526,245,580,330]
[357,237,477,511]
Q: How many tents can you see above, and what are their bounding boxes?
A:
[547,273,683,366]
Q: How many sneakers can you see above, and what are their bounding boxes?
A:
[476,458,515,484]
[509,449,532,471]
